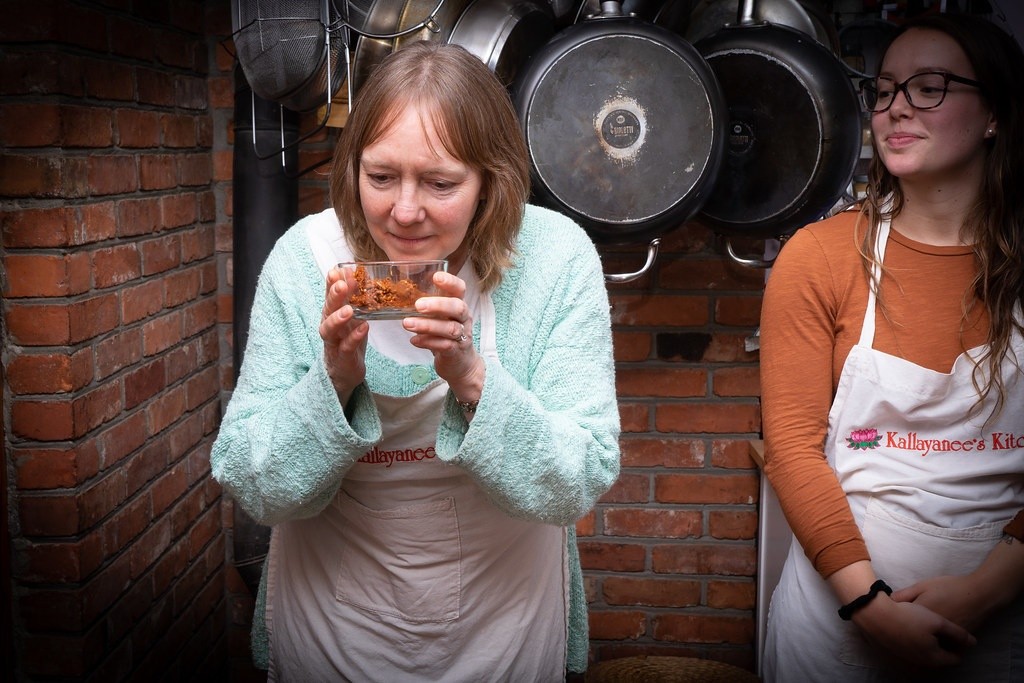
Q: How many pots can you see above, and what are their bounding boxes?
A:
[514,0,721,283]
[341,0,540,96]
[687,0,861,266]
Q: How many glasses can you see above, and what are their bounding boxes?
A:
[858,69,988,113]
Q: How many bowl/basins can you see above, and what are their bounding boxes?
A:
[339,259,450,321]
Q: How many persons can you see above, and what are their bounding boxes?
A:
[209,40,621,683]
[759,13,1024,683]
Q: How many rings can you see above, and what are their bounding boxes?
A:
[456,325,467,341]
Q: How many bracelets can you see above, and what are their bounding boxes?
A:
[454,397,480,413]
[838,579,893,620]
